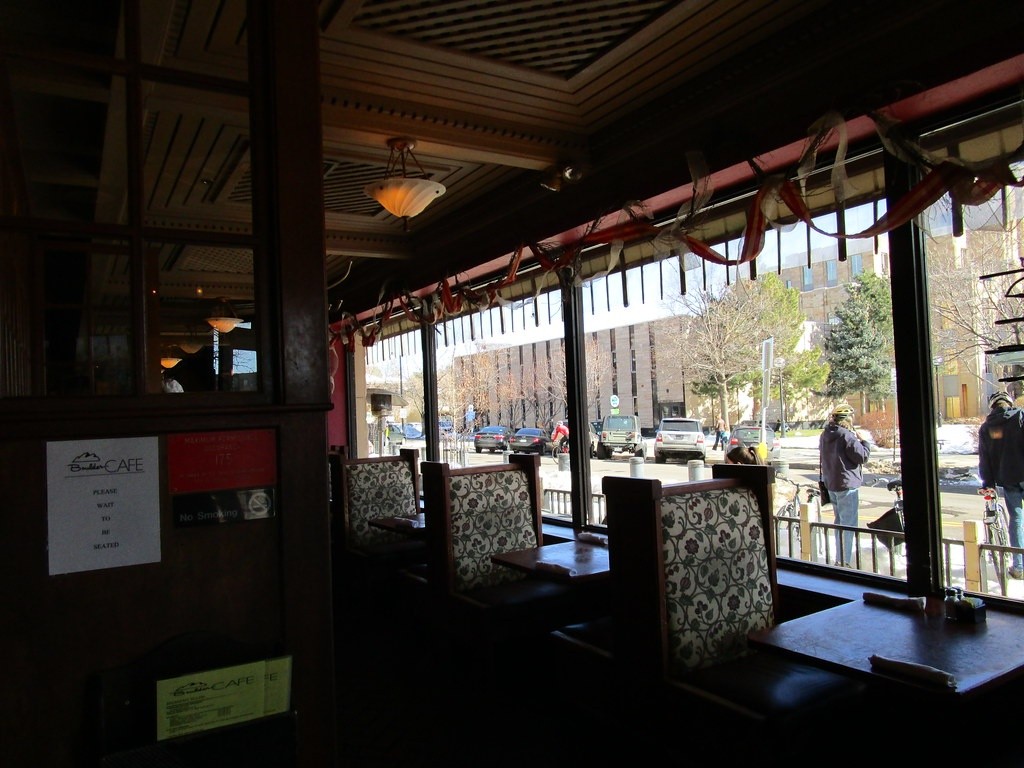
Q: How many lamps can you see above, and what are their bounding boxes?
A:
[560,160,585,184]
[365,136,446,231]
[161,344,183,369]
[177,323,205,354]
[206,296,244,342]
[540,169,561,192]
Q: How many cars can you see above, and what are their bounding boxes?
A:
[653,418,706,464]
[474,426,516,453]
[510,427,553,456]
[935,425,984,488]
[551,420,600,458]
[388,420,453,438]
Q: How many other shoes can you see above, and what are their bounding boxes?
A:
[1009,566,1024,579]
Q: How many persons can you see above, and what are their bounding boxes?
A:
[727,446,765,465]
[759,419,793,432]
[712,415,726,451]
[552,421,569,454]
[978,391,1024,581]
[818,404,871,569]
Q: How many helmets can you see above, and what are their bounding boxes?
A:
[989,392,1013,411]
[831,405,855,424]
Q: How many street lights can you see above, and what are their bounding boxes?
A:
[775,357,786,438]
[932,354,943,428]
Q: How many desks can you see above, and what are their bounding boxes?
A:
[491,541,611,584]
[745,590,1024,699]
[368,512,427,541]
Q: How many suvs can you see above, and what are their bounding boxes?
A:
[720,423,781,463]
[596,415,649,459]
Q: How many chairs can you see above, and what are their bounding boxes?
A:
[331,447,864,768]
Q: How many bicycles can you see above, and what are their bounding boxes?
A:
[977,485,1012,587]
[888,477,906,557]
[551,440,571,463]
[777,479,821,542]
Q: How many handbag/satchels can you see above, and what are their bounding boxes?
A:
[818,481,832,507]
